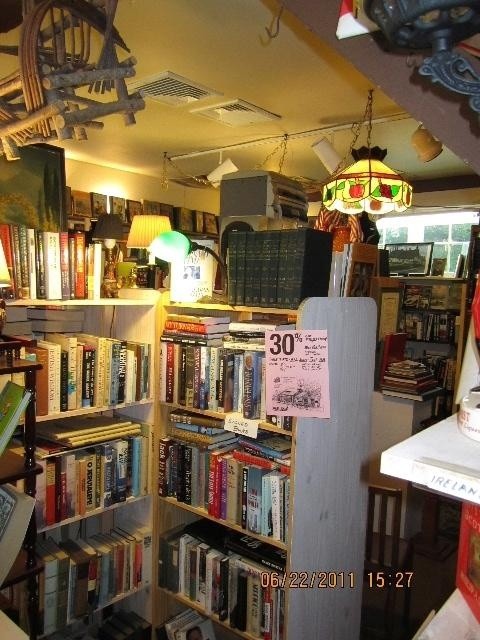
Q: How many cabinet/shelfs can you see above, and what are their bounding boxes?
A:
[395,278,470,417]
[0,292,378,640]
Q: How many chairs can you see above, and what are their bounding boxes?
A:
[359,484,413,640]
[1,1,146,161]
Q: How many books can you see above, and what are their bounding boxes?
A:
[228,227,380,310]
[1,307,152,639]
[376,284,463,417]
[154,312,294,639]
[0,224,102,299]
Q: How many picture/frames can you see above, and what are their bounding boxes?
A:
[384,242,434,277]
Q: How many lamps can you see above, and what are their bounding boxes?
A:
[207,125,442,189]
[149,230,228,305]
[92,214,122,299]
[126,215,172,270]
[322,146,413,215]
